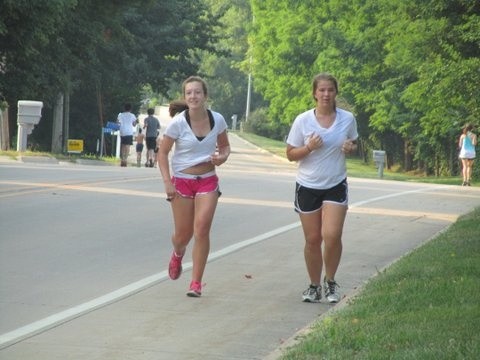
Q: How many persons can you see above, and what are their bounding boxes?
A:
[118,105,161,168]
[287,74,359,304]
[158,76,232,297]
[458,123,476,186]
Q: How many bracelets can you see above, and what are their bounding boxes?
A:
[305,143,312,153]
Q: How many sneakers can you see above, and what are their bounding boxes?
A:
[323,276,338,302]
[168,250,183,279]
[187,281,201,297]
[303,285,321,303]
